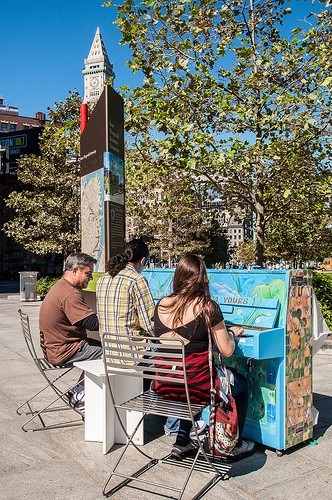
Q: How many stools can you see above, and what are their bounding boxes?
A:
[73,358,145,454]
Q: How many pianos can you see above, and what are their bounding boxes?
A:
[138,266,319,457]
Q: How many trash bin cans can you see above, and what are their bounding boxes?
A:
[18,271,39,302]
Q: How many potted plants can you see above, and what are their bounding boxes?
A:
[35,277,52,301]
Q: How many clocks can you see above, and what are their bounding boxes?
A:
[90,78,99,87]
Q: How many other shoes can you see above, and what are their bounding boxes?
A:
[171,438,203,460]
[222,440,256,463]
[165,420,206,437]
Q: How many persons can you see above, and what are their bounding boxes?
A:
[96,239,206,436]
[151,254,256,463]
[39,253,103,414]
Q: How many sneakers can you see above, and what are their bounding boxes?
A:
[65,382,85,418]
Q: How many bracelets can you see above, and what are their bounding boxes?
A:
[227,329,235,335]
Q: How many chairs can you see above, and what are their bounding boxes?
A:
[16,309,85,433]
[101,332,233,500]
